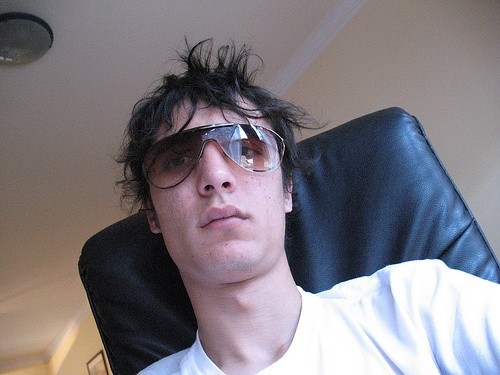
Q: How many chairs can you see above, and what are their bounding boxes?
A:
[77,106,499,375]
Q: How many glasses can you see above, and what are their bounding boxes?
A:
[141,123,286,189]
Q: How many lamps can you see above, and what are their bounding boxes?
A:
[0,13,54,65]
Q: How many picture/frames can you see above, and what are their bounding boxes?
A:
[86,350,110,375]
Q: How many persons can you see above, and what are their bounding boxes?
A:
[113,36,500,375]
